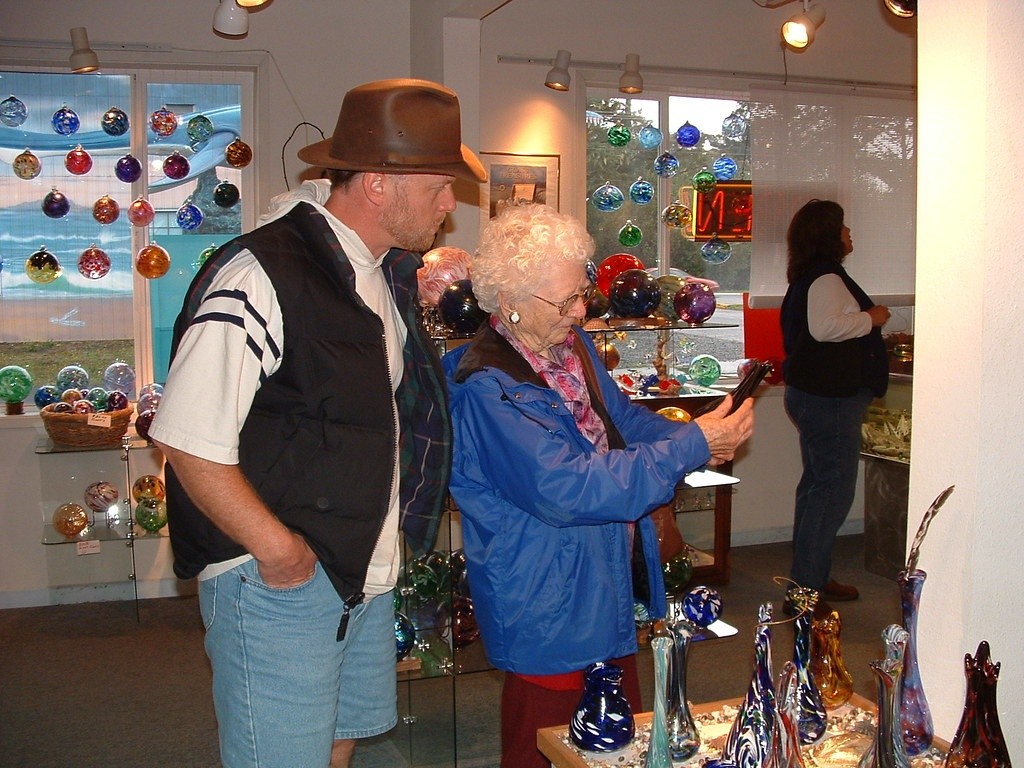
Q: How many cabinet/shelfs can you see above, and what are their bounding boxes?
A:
[608,324,740,588]
[397,494,740,768]
[35,433,176,626]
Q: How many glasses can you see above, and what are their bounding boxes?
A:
[530,280,597,316]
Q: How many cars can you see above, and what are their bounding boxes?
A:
[646,267,720,292]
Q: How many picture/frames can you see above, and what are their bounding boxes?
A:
[479,151,561,232]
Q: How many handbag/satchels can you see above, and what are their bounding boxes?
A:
[571,332,685,561]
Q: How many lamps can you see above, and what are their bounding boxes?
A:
[544,49,574,92]
[68,26,99,74]
[214,0,250,38]
[782,3,827,55]
[617,53,644,92]
[885,0,917,18]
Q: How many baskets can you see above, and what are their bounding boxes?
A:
[41,402,134,445]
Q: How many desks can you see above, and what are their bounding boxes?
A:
[533,688,954,768]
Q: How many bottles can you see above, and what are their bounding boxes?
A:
[569,569,1012,768]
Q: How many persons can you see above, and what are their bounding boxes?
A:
[440,204,755,768]
[781,201,889,622]
[146,78,487,768]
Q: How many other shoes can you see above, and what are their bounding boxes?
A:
[782,593,832,621]
[822,579,858,601]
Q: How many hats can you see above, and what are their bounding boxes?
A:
[298,79,487,182]
